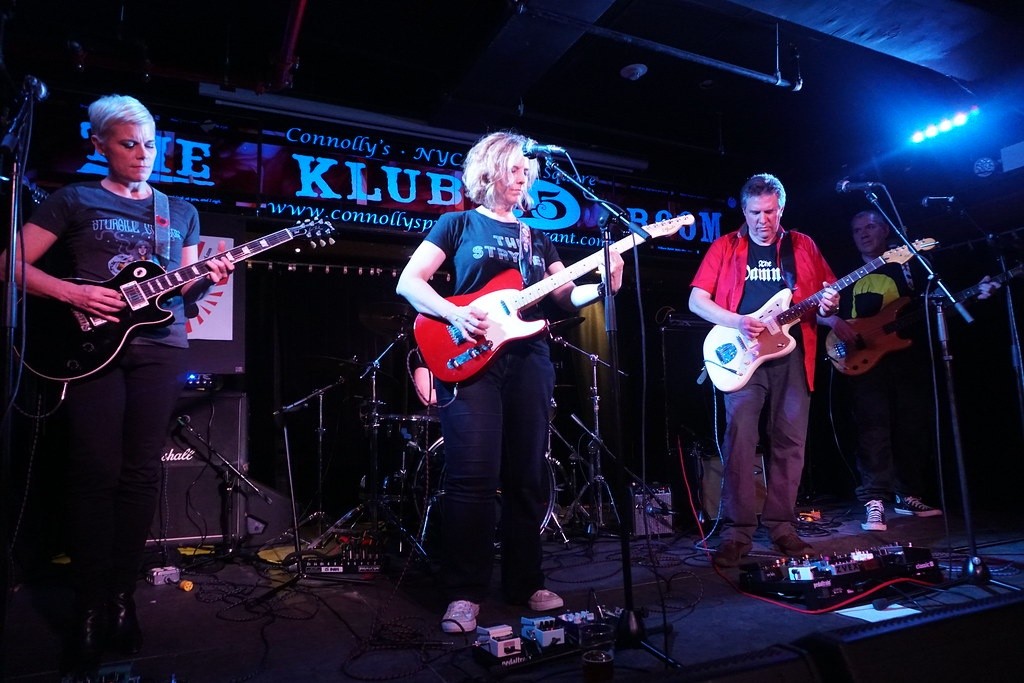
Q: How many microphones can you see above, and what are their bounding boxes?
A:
[922,194,958,207]
[26,75,47,101]
[836,180,881,193]
[645,505,680,517]
[177,415,191,423]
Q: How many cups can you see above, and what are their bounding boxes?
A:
[579,624,615,664]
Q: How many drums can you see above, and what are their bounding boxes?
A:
[411,435,557,560]
[358,414,442,504]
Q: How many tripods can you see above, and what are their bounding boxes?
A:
[488,154,689,683]
[866,192,1024,611]
[242,325,430,611]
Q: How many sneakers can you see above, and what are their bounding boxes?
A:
[770,532,816,556]
[517,589,564,611]
[442,600,480,632]
[862,500,888,531]
[714,538,752,565]
[894,496,942,516]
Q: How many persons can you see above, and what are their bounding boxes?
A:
[688,173,841,566]
[815,211,1000,528]
[0,95,235,656]
[395,131,625,633]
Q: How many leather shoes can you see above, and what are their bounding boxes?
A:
[111,594,144,656]
[68,603,109,659]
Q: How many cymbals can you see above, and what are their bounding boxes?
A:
[546,317,586,334]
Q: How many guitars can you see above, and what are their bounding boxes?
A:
[825,264,1024,376]
[413,214,695,383]
[14,217,336,393]
[703,238,936,394]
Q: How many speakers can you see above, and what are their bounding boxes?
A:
[626,486,672,538]
[660,325,728,531]
[145,392,248,546]
[646,642,823,683]
[235,476,300,545]
[791,588,1024,683]
